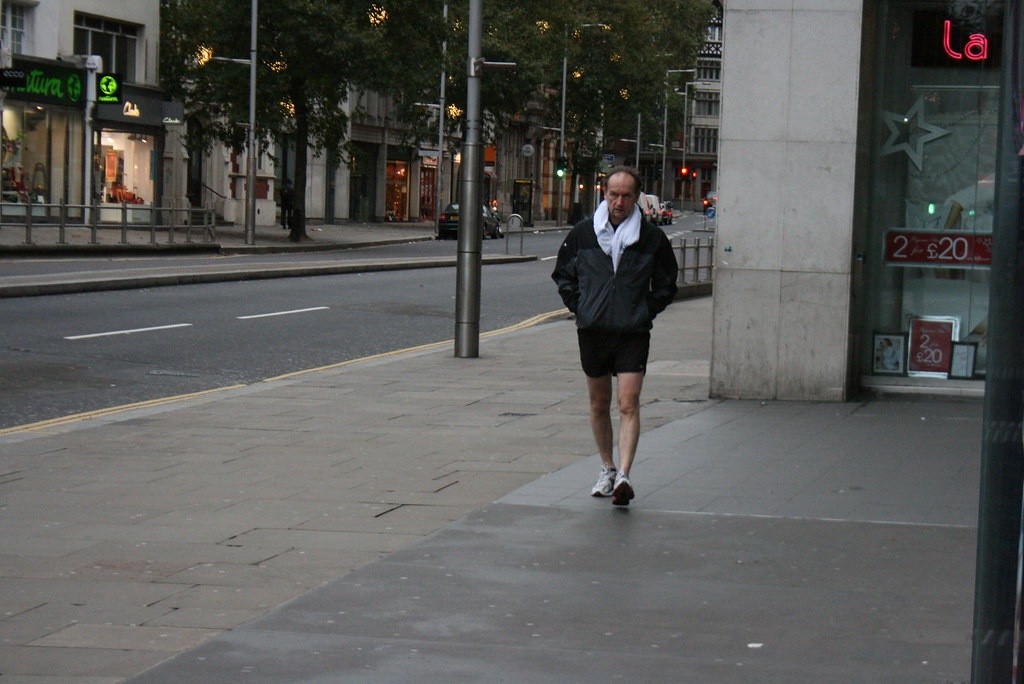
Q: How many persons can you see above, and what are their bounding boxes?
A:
[550,165,678,506]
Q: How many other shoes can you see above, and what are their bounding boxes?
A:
[288,226,290,228]
[283,225,286,229]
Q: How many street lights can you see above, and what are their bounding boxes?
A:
[211,57,256,246]
[412,102,445,219]
[681,82,712,213]
[556,21,610,226]
[661,69,696,201]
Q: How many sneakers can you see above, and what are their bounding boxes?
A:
[590,465,618,497]
[612,472,635,506]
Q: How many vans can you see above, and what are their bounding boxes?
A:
[637,193,653,223]
[646,195,664,225]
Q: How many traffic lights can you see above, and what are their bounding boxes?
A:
[554,156,565,176]
[680,167,688,179]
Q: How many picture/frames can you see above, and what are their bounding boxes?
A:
[947,341,979,381]
[872,332,909,377]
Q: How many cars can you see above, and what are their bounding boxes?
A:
[437,200,504,240]
[660,202,674,224]
[701,191,716,215]
